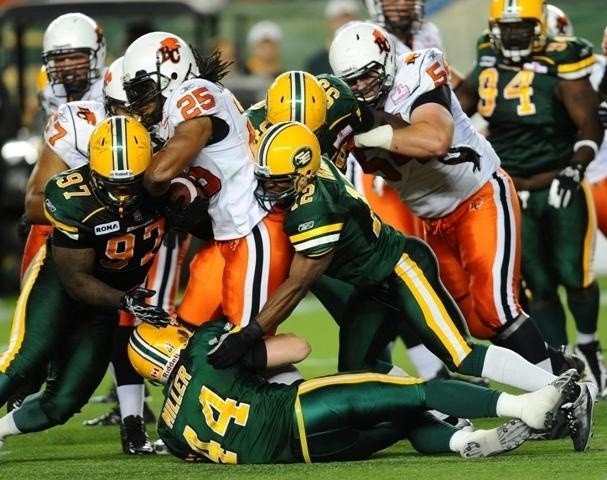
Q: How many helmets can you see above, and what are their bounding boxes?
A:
[264,70,328,139]
[488,1,547,63]
[544,4,572,39]
[126,316,196,387]
[315,72,362,148]
[42,12,202,218]
[365,0,428,38]
[251,121,322,215]
[328,24,396,114]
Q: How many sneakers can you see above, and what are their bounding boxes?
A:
[83,402,157,427]
[89,379,153,406]
[119,414,154,456]
[428,339,606,461]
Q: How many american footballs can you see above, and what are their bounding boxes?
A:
[168,175,204,208]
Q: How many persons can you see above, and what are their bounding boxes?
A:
[1,1,607,464]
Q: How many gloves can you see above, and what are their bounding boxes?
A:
[232,343,268,385]
[120,288,172,330]
[439,145,482,174]
[207,318,265,371]
[547,162,587,212]
